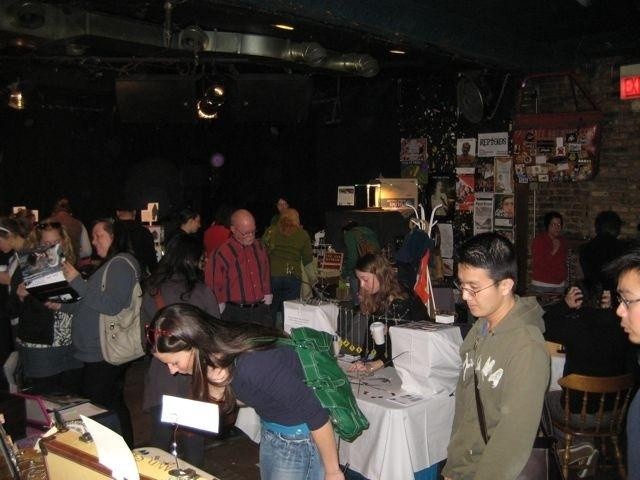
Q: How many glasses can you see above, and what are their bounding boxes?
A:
[453,277,503,297]
[616,296,640,310]
[550,222,561,227]
[236,228,255,237]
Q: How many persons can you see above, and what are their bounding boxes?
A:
[264,209,314,310]
[17,220,81,395]
[163,208,201,252]
[145,301,371,479]
[211,209,272,325]
[112,202,155,263]
[440,232,552,479]
[338,216,378,292]
[457,142,472,168]
[142,233,220,321]
[530,213,568,311]
[602,253,640,479]
[498,198,513,219]
[204,214,230,262]
[46,219,142,448]
[42,198,91,265]
[0,214,31,314]
[270,198,288,224]
[542,276,629,479]
[581,211,638,292]
[349,252,429,375]
[431,180,447,215]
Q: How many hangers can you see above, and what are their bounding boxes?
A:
[405,203,442,318]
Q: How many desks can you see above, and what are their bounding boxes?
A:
[93,407,122,446]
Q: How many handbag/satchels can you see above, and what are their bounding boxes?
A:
[99,256,147,365]
[240,327,370,443]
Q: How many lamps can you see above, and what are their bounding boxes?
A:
[161,394,219,478]
[457,71,493,125]
[198,86,224,119]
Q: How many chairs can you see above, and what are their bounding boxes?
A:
[552,375,639,480]
